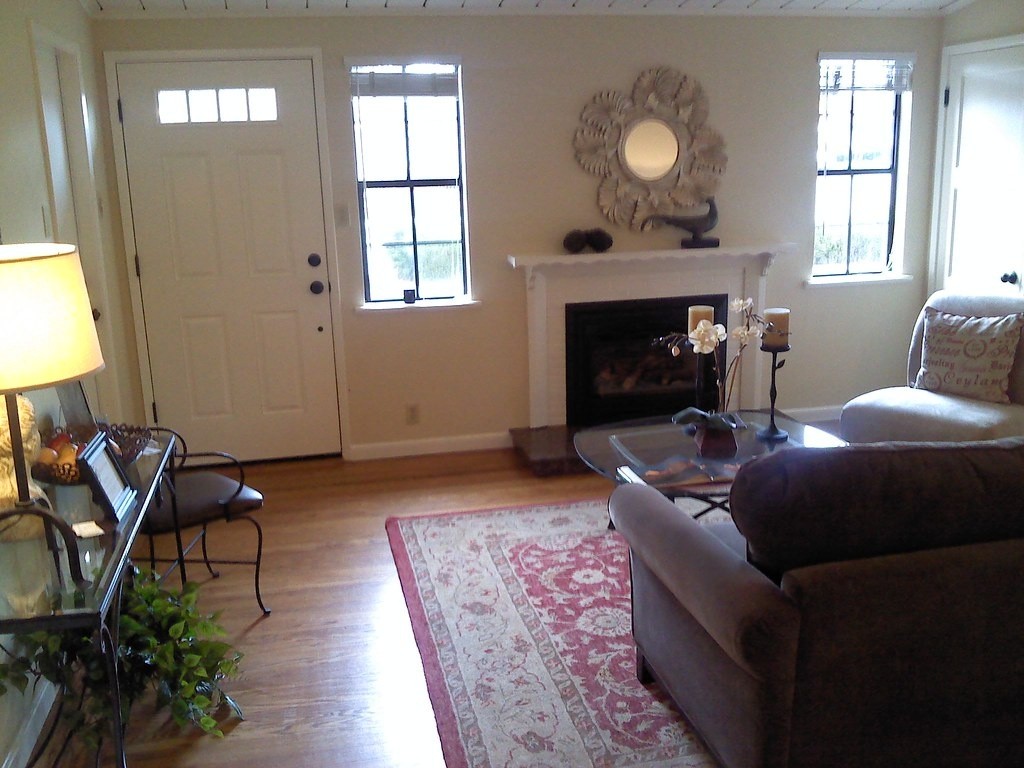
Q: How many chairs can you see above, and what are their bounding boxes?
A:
[130,426,273,614]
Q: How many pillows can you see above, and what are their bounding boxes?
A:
[914,306,1024,403]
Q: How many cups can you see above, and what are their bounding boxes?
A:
[404,290,415,303]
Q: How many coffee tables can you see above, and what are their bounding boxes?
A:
[573,413,851,520]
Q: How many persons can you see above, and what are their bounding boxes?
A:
[0,393,57,541]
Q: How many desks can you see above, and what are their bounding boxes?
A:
[0,431,176,768]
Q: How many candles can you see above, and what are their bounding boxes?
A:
[687,305,714,338]
[763,309,791,346]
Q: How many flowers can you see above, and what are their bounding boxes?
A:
[651,298,764,409]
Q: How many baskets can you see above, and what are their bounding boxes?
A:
[31,421,152,486]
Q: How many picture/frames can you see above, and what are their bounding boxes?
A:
[78,431,136,522]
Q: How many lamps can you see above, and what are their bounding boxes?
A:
[0,241,105,587]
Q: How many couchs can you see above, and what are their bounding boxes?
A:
[838,293,1024,441]
[609,443,1024,768]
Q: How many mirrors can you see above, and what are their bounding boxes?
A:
[574,70,728,233]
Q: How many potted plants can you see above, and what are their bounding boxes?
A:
[0,569,242,768]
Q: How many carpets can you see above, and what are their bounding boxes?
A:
[385,480,734,768]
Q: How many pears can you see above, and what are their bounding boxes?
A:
[40,442,77,477]
